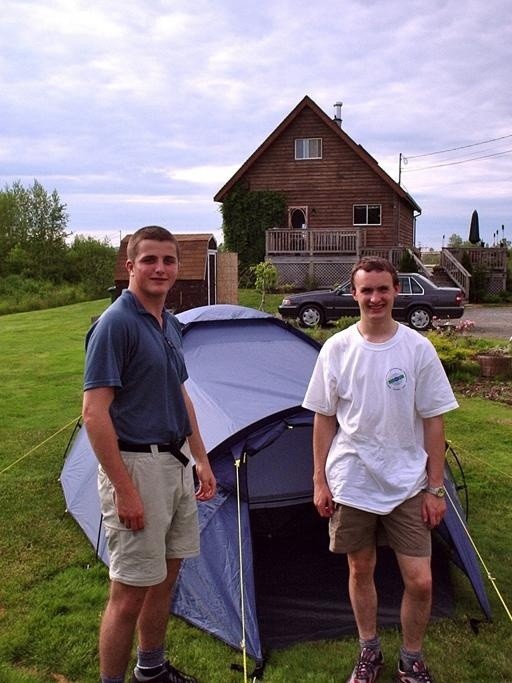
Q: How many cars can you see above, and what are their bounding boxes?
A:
[278,270,468,331]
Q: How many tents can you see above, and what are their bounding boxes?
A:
[58,303,495,680]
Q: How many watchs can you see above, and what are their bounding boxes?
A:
[424,485,446,498]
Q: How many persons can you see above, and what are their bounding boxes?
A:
[80,225,218,683]
[301,254,461,683]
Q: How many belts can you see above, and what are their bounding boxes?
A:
[119,438,190,468]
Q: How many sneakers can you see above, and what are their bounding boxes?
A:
[348,648,385,682]
[396,659,432,683]
[131,659,198,683]
